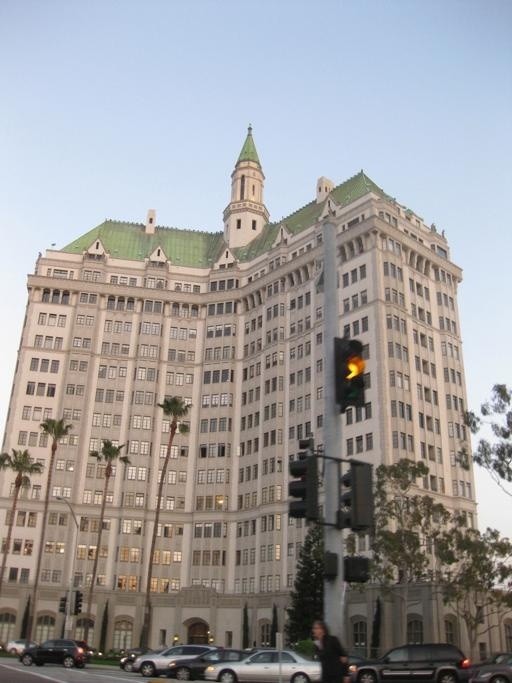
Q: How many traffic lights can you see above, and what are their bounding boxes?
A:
[73,590,83,615]
[333,336,366,409]
[288,453,320,522]
[341,458,376,533]
[322,550,338,578]
[59,596,66,612]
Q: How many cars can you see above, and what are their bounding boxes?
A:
[343,655,377,677]
[468,652,512,683]
[119,644,322,683]
[5,639,40,658]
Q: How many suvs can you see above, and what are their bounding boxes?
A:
[347,642,473,683]
[19,638,91,669]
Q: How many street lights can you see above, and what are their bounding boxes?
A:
[55,495,80,640]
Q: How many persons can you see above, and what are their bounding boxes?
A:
[310,617,353,683]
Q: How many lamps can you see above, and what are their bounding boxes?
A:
[210,635,214,642]
[173,634,180,646]
